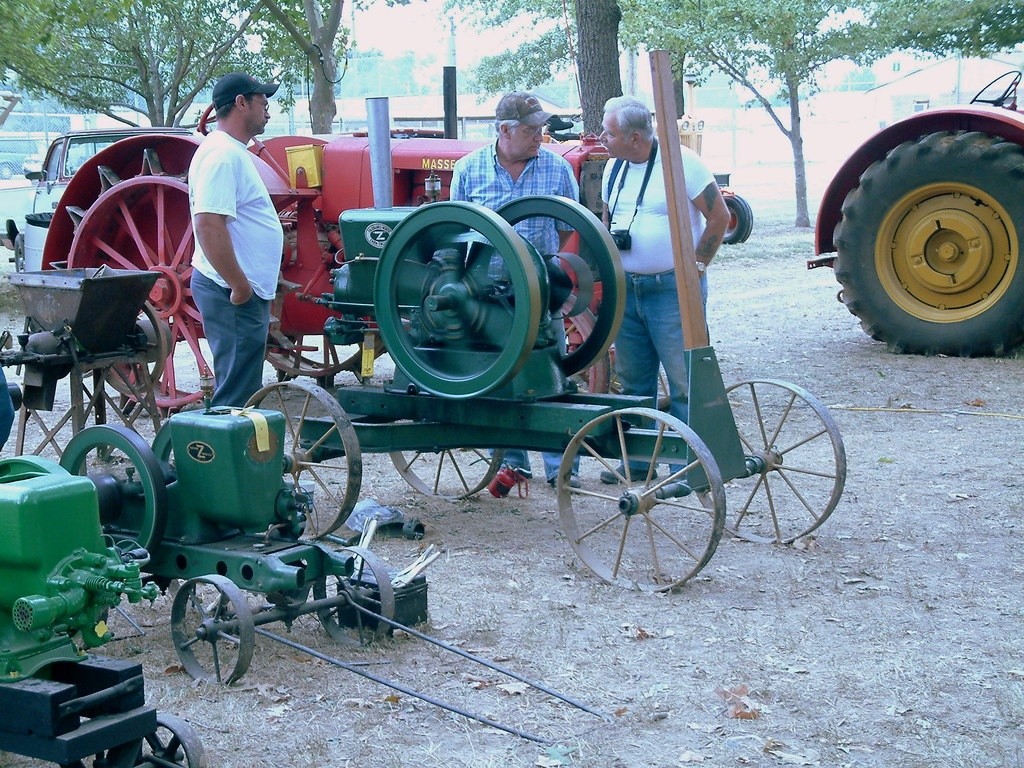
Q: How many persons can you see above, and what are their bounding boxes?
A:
[601,95,730,485]
[451,91,582,490]
[189,75,285,405]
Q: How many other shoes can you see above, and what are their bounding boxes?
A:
[664,472,687,486]
[553,473,581,494]
[600,464,658,485]
[497,461,532,480]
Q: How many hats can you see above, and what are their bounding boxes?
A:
[212,72,280,110]
[496,90,558,126]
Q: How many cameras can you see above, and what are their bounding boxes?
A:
[611,229,632,251]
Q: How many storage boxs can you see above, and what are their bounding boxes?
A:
[285,144,323,188]
[336,573,429,632]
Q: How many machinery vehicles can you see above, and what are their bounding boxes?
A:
[40,66,664,419]
[804,71,1023,360]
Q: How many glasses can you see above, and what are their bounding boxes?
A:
[234,96,270,112]
[517,122,550,136]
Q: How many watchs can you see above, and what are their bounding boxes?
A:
[696,262,706,271]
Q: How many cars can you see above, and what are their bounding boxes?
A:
[0,131,59,181]
[26,128,193,216]
